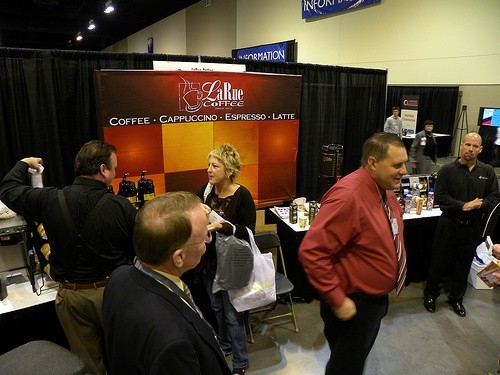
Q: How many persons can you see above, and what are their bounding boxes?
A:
[196,143,256,375]
[0,140,137,375]
[409,120,438,173]
[297,131,408,375]
[424,132,500,318]
[102,192,233,375]
[384,107,402,140]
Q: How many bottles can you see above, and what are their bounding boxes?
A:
[309,201,316,225]
[426,188,434,210]
[404,189,412,213]
[289,203,298,224]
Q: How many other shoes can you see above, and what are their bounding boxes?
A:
[222,348,233,355]
[231,364,249,375]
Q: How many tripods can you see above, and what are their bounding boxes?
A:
[445,109,468,159]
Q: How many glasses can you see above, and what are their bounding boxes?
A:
[174,230,212,253]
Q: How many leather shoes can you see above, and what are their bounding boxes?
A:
[424,297,436,313]
[451,300,466,317]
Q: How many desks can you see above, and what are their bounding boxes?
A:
[265,197,443,303]
[402,133,452,157]
[0,276,72,353]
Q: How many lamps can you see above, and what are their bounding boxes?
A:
[103,0,115,14]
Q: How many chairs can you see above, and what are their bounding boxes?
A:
[243,232,299,343]
[0,340,86,375]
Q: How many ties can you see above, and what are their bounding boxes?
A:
[183,282,194,308]
[382,191,407,297]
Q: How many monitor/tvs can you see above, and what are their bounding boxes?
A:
[477,106,500,127]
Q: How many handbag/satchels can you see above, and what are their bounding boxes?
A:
[228,227,276,312]
[211,220,254,294]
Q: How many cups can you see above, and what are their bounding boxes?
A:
[299,217,307,228]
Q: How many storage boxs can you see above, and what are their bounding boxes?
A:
[467,261,494,290]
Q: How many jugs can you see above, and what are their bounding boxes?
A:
[118,170,155,210]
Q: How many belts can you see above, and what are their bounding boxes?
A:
[59,281,107,289]
